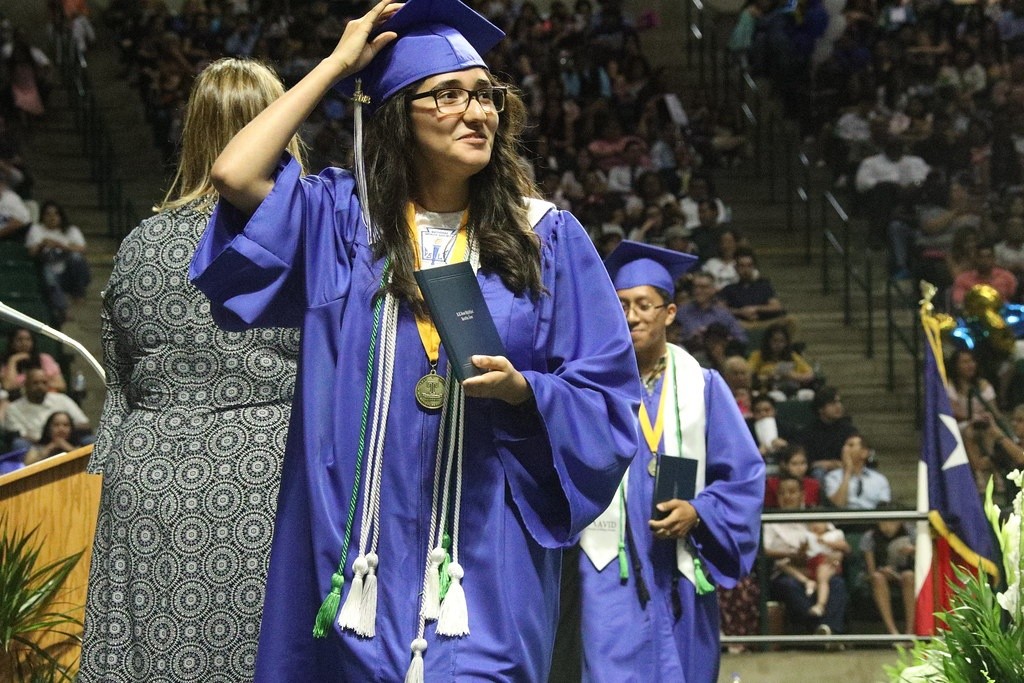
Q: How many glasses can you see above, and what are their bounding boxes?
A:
[408,86,507,115]
[621,300,667,316]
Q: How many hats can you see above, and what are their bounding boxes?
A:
[602,239,698,304]
[331,0,507,245]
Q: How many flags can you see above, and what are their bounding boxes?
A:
[916,292,1008,645]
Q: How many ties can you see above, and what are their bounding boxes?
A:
[856,480,862,496]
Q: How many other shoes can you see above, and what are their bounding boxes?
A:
[803,579,815,597]
[812,624,846,652]
[809,606,824,617]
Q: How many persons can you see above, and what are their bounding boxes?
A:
[2,1,1023,682]
[188,0,643,683]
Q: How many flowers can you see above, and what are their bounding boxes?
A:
[882,467,1024,683]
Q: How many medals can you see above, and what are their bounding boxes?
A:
[414,369,453,413]
